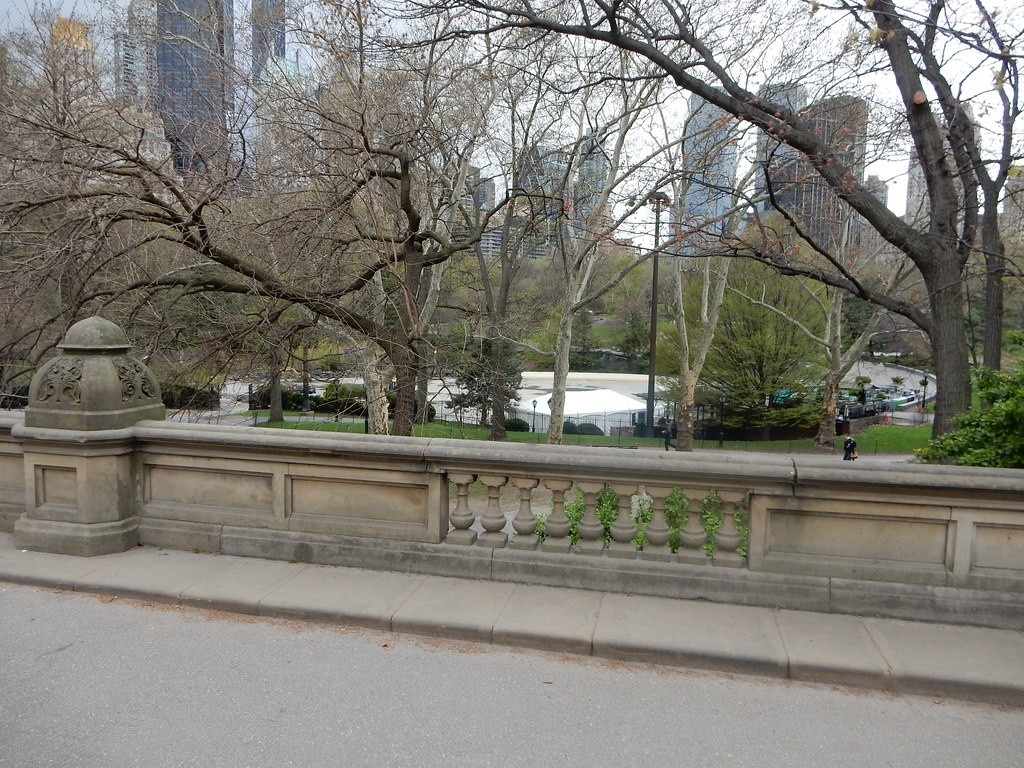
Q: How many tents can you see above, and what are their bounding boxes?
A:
[512,387,651,433]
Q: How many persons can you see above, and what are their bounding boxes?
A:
[661,423,677,451]
[843,436,859,462]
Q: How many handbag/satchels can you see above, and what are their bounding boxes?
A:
[851,451,858,459]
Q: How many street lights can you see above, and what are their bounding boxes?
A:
[644,191,670,437]
[334,378,340,422]
[922,369,929,407]
[532,400,537,432]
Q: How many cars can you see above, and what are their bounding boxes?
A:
[287,384,316,394]
[281,368,309,381]
